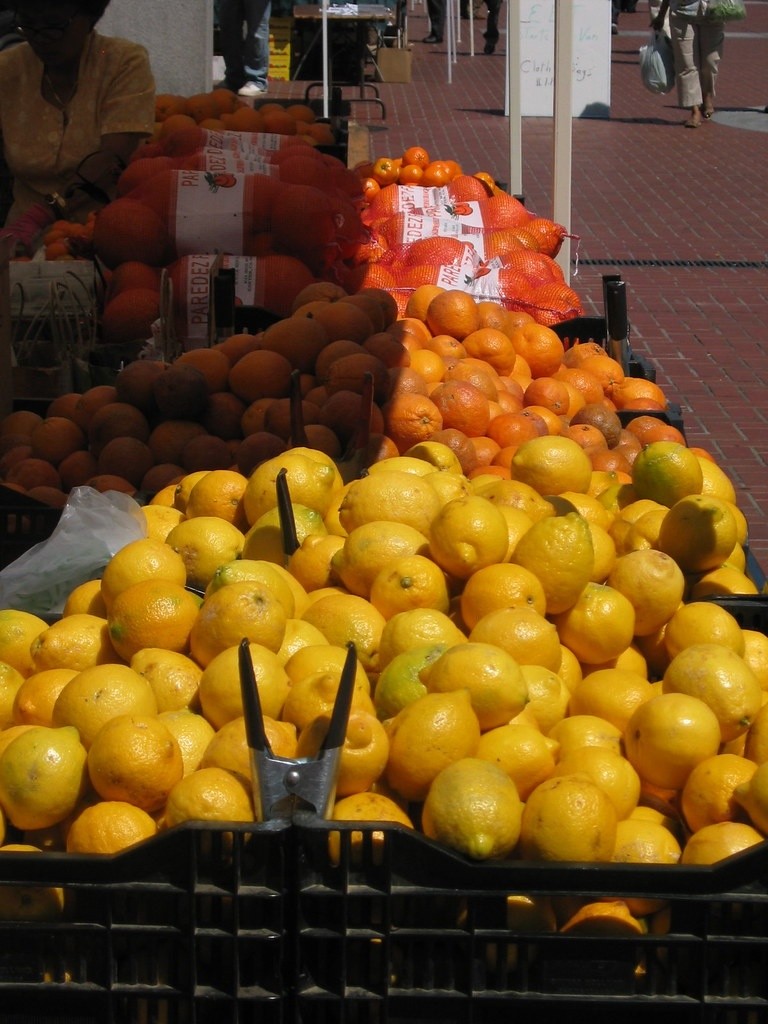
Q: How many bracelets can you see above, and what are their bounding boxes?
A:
[45,192,70,220]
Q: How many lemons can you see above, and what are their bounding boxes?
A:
[1,439,768,994]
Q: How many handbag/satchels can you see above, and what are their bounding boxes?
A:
[638,25,675,98]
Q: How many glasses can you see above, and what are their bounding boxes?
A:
[7,7,80,44]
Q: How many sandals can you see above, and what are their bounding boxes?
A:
[700,103,713,119]
[686,119,701,129]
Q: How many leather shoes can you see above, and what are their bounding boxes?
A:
[421,33,442,43]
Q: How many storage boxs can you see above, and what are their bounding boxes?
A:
[203,262,687,440]
[0,594,768,1023]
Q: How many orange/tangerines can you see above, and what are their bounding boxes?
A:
[1,88,720,529]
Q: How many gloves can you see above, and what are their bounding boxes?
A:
[0,201,55,257]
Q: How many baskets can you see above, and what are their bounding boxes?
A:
[0,818,766,1023]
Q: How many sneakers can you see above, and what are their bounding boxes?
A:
[237,83,268,97]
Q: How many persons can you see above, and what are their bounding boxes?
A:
[215,0,272,97]
[1,1,158,254]
[422,0,447,44]
[480,0,504,56]
[649,0,726,129]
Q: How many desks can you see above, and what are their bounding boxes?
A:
[293,4,393,121]
